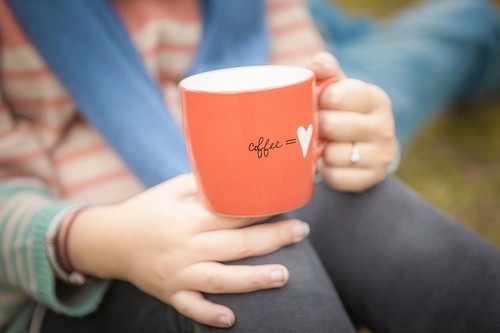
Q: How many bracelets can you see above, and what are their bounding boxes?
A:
[43,201,86,285]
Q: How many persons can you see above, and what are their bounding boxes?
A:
[0,0,500,333]
[308,1,500,141]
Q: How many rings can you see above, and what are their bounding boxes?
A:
[351,144,361,164]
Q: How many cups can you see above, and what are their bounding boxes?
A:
[178,64,331,219]
